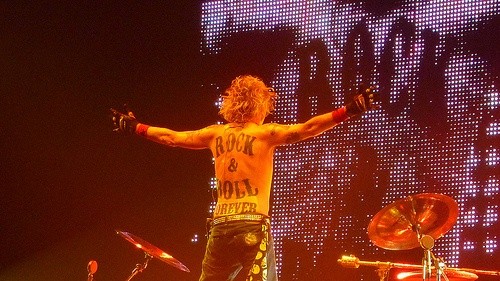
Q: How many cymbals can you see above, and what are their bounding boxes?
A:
[367,192,459,250]
[113,224,191,272]
[396,272,479,281]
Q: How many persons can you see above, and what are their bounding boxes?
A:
[109,75,381,281]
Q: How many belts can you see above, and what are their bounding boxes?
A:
[210,214,263,225]
